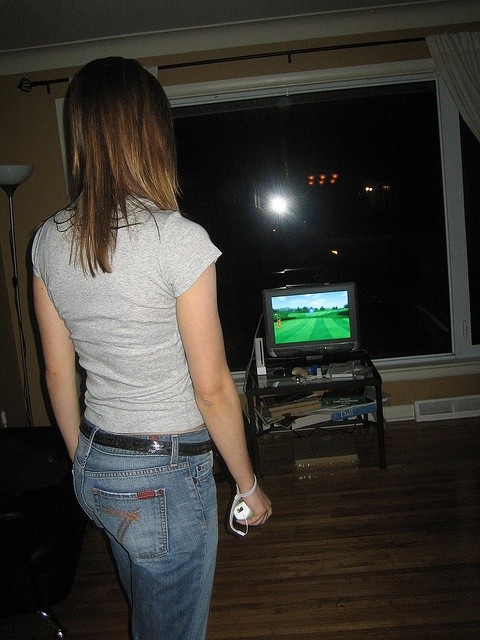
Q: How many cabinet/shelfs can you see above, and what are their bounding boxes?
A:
[244,349,389,479]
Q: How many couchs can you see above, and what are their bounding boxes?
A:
[0,426,89,617]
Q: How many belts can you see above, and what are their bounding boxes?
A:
[78,415,216,457]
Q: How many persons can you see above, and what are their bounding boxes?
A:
[32,58,273,640]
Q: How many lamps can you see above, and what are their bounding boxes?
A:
[0,163,34,425]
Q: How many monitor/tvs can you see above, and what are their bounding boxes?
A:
[264,282,362,360]
